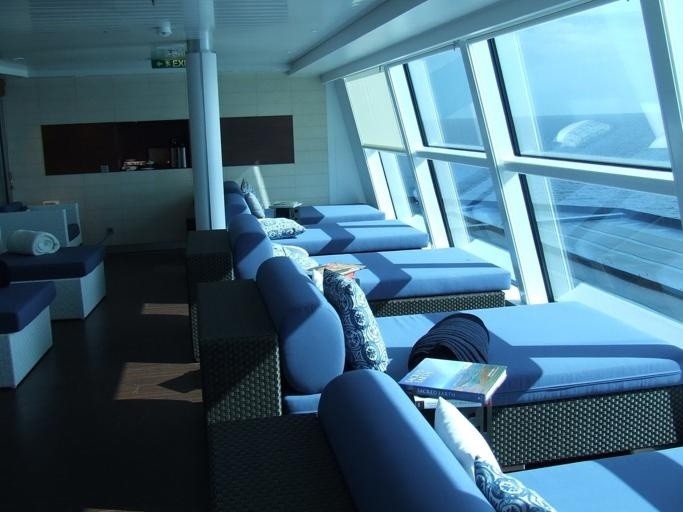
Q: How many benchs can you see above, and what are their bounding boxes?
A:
[1,247,109,390]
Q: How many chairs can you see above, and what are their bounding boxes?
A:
[222,256,682,473]
[220,181,512,318]
[315,366,682,512]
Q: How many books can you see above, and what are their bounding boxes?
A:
[305,262,367,280]
[268,201,303,208]
[397,358,509,409]
